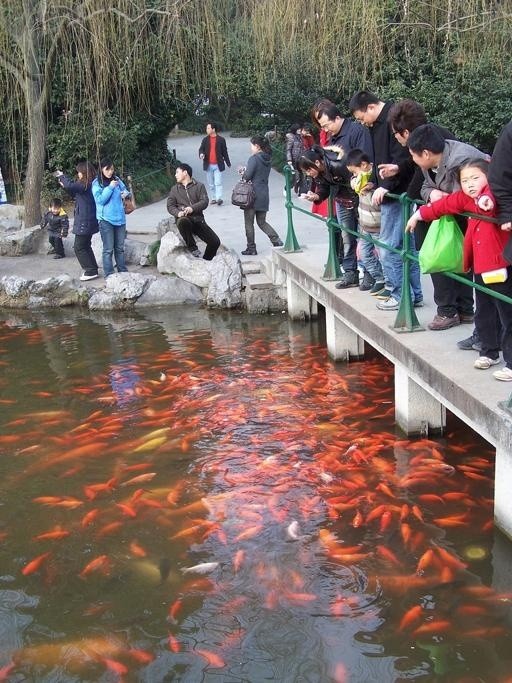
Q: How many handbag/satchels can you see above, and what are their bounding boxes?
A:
[122,193,135,214]
[232,180,256,208]
[418,214,465,275]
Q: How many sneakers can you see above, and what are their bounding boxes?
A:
[48,249,56,253]
[210,200,215,204]
[298,193,307,198]
[53,253,64,258]
[458,328,480,349]
[474,355,501,369]
[472,340,483,350]
[80,272,97,280]
[190,247,201,256]
[459,311,474,323]
[282,186,287,196]
[377,296,401,309]
[494,366,512,381]
[369,280,385,295]
[217,198,223,205]
[428,314,460,330]
[376,289,391,299]
[412,298,423,306]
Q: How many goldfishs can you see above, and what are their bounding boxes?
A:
[1,304,511,682]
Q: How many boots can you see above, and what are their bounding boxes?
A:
[241,244,257,255]
[359,271,375,290]
[335,269,360,288]
[272,238,283,246]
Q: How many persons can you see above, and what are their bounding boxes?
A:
[310,97,343,268]
[348,90,425,311]
[316,103,377,289]
[198,122,231,205]
[166,163,221,260]
[41,198,70,259]
[374,97,475,331]
[299,126,314,192]
[92,157,133,280]
[56,161,100,281]
[403,155,511,382]
[485,116,511,273]
[406,123,494,351]
[241,134,284,255]
[294,145,364,290]
[343,148,395,301]
[283,123,308,197]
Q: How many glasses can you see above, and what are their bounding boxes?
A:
[392,131,398,136]
[321,122,333,129]
